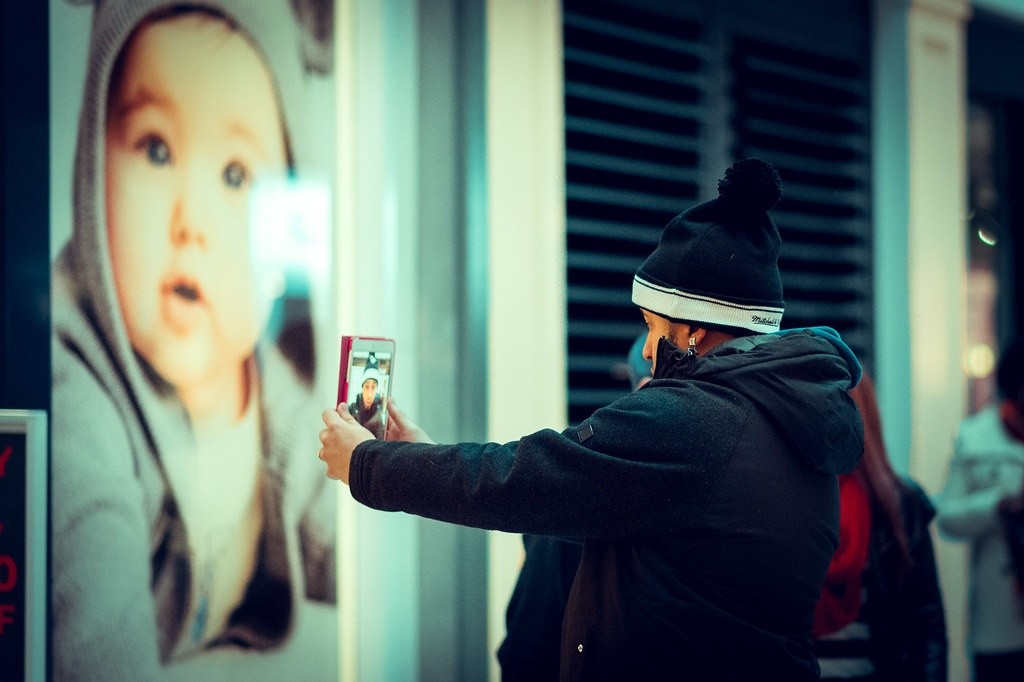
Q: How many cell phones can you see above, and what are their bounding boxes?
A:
[346,336,396,441]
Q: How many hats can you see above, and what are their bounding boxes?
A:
[361,352,380,387]
[632,158,786,333]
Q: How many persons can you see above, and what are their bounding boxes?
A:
[320,159,865,682]
[934,336,1024,682]
[48,0,335,681]
[813,374,948,682]
[349,352,384,441]
